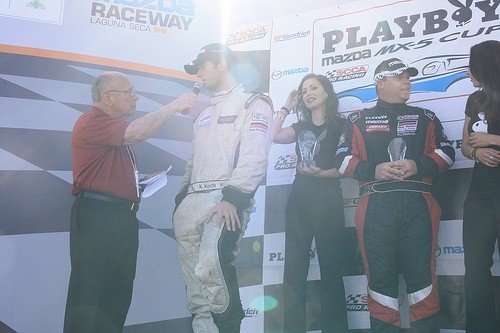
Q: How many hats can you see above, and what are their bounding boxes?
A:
[184,43,236,75]
[374,58,418,86]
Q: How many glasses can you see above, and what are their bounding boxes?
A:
[107,89,136,97]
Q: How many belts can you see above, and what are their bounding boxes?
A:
[78,192,139,212]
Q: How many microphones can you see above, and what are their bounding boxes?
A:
[182,81,202,115]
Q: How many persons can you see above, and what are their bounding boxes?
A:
[273,73,351,333]
[172,43,276,333]
[461,40,500,332]
[335,58,456,333]
[63,72,200,333]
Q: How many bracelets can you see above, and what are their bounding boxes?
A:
[470,147,480,163]
[274,114,285,121]
[157,107,166,119]
[281,107,290,115]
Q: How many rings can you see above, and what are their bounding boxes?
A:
[190,105,192,107]
[489,156,494,160]
